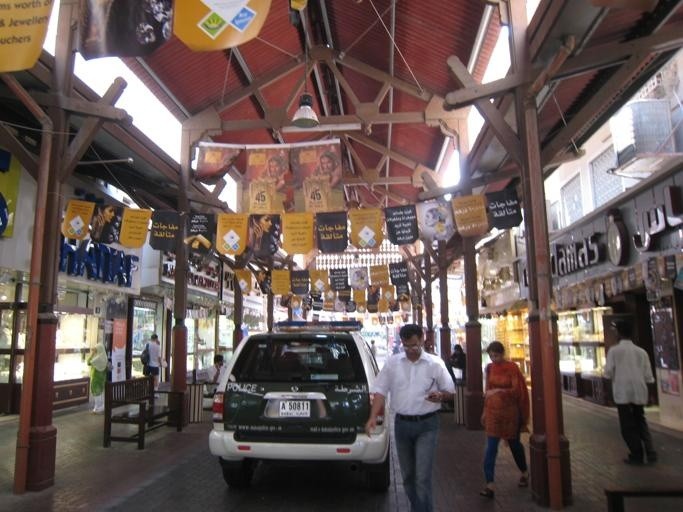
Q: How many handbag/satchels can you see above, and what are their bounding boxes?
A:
[140,349,150,375]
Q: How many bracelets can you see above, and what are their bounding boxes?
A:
[437,390,444,403]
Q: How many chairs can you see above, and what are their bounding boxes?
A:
[271,351,346,374]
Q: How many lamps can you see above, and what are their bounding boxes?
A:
[290,7,319,128]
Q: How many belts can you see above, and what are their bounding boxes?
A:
[395,412,436,421]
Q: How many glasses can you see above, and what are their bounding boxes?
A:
[401,344,423,351]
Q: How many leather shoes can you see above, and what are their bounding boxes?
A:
[518,472,529,487]
[479,487,495,498]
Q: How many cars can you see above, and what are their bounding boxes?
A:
[207,319,391,498]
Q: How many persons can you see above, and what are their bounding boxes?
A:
[362,323,459,510]
[423,339,436,356]
[141,334,163,399]
[368,337,376,357]
[476,339,531,499]
[448,342,466,381]
[90,205,116,243]
[425,208,454,241]
[258,156,291,200]
[310,150,342,188]
[249,214,273,249]
[369,286,376,295]
[255,271,266,286]
[204,353,226,395]
[86,342,109,415]
[599,318,658,467]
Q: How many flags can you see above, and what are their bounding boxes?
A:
[0,1,55,71]
[175,0,276,56]
[68,1,174,64]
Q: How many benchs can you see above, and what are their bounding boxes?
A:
[103,368,219,450]
